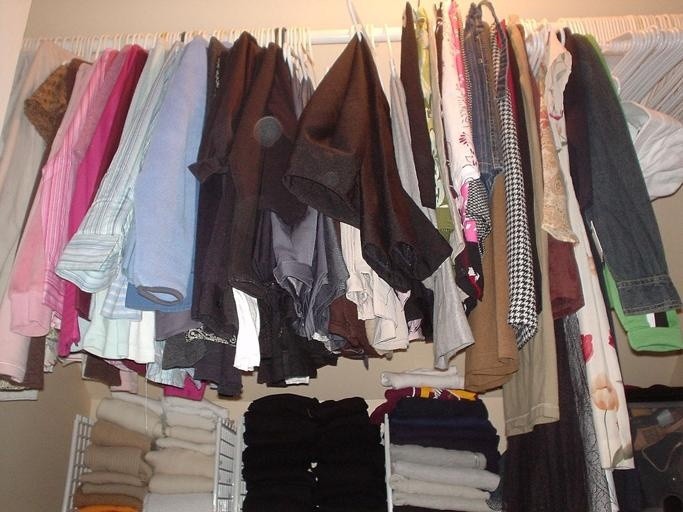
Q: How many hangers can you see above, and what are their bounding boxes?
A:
[19,23,314,83]
